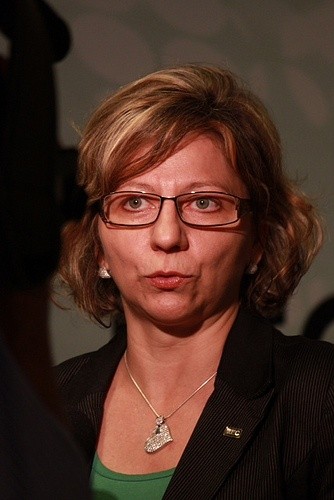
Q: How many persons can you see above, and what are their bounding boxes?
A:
[0,61,334,500]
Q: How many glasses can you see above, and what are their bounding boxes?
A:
[93,191,259,227]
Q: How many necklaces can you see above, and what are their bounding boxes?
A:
[122,349,217,454]
